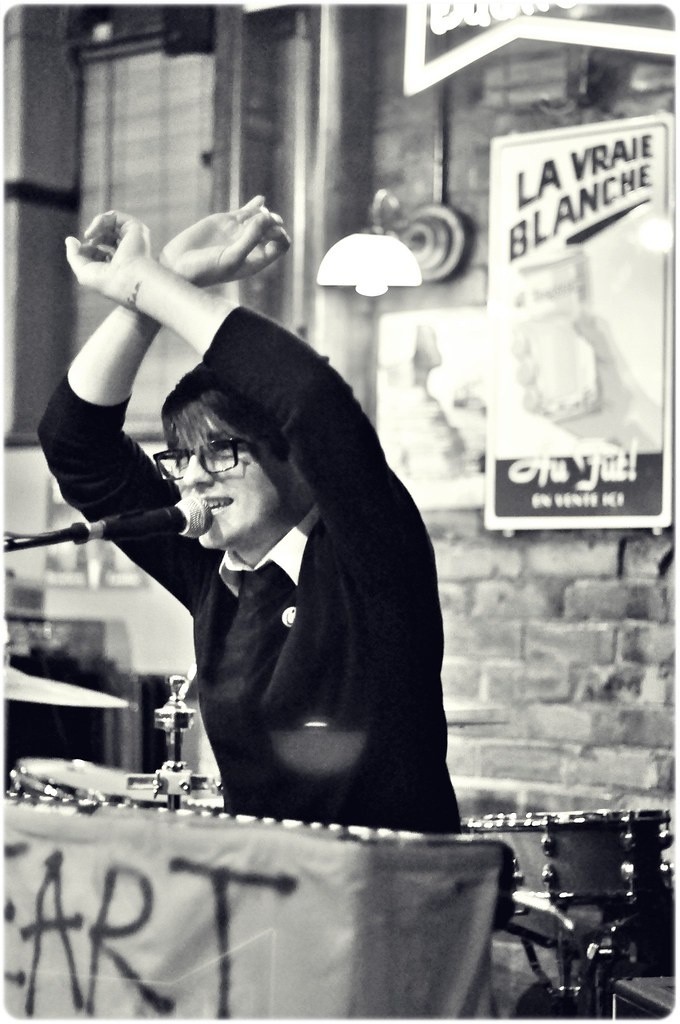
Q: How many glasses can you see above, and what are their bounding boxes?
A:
[152,437,245,482]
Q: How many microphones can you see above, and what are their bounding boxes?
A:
[70,497,213,545]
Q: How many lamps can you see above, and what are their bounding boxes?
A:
[317,190,467,298]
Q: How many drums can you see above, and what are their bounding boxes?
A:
[465,802,675,905]
[7,749,225,814]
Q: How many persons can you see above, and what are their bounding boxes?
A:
[38,194,463,835]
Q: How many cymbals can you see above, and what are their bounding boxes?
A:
[5,664,141,712]
